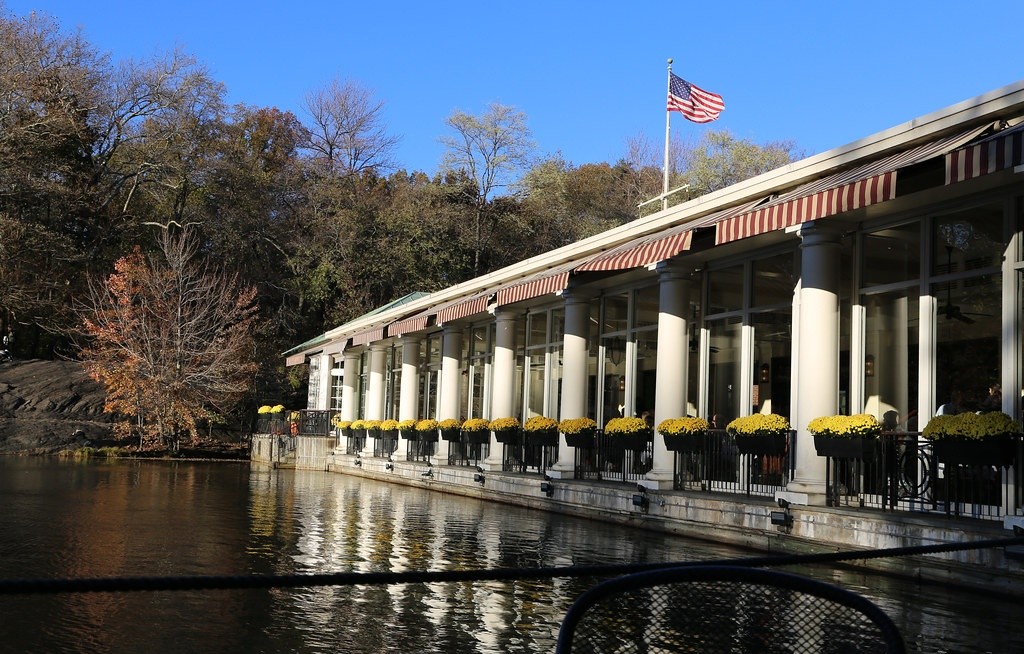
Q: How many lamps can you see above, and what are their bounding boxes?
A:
[866,354,874,376]
[759,363,769,382]
[610,321,627,368]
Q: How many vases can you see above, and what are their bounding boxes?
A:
[271,411,285,419]
[932,433,1013,466]
[812,435,875,458]
[529,428,557,446]
[662,432,706,453]
[735,431,786,455]
[259,412,272,419]
[340,428,523,444]
[564,428,594,446]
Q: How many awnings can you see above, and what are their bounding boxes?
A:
[279,112,1024,369]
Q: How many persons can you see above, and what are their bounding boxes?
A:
[641,410,654,474]
[936,382,1002,417]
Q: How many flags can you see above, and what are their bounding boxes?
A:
[667,70,725,123]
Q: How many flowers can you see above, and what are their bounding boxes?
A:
[437,418,463,432]
[258,405,273,415]
[805,412,883,439]
[918,410,1023,442]
[655,417,710,436]
[724,412,789,435]
[349,419,369,431]
[364,420,382,430]
[487,417,520,432]
[397,420,419,431]
[524,415,559,433]
[460,418,491,433]
[415,418,438,433]
[269,404,286,414]
[556,417,599,435]
[379,418,400,431]
[335,421,353,430]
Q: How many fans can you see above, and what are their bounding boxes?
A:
[906,241,995,325]
[687,301,721,354]
